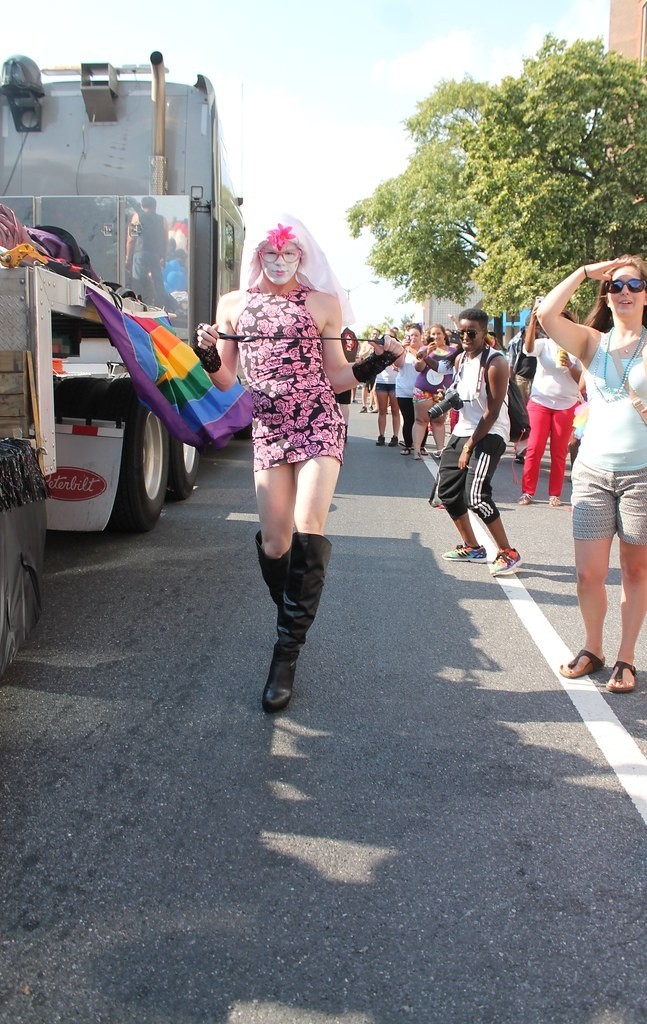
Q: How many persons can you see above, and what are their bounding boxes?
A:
[125,197,189,318]
[537,255,647,691]
[508,313,548,463]
[518,306,581,506]
[415,309,522,576]
[334,328,361,444]
[352,315,463,458]
[194,215,406,714]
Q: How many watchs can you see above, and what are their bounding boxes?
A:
[463,445,471,452]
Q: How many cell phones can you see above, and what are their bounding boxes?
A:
[632,399,647,425]
[405,335,410,340]
[535,296,544,308]
[427,338,437,350]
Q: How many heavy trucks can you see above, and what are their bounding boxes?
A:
[1,38,247,537]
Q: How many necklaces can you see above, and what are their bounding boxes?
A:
[594,325,647,402]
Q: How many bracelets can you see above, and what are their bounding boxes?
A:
[585,267,587,277]
[569,363,575,369]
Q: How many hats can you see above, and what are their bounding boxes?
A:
[562,306,577,323]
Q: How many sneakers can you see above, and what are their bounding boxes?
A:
[442,541,488,564]
[490,547,523,576]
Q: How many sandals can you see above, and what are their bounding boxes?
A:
[400,447,411,455]
[549,495,562,508]
[420,447,428,456]
[517,493,533,506]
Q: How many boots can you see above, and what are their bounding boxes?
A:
[261,531,332,715]
[254,527,291,607]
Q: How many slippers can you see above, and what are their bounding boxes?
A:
[606,661,637,692]
[559,650,605,679]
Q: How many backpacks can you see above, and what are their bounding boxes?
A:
[486,352,531,443]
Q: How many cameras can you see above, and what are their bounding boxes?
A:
[427,390,463,419]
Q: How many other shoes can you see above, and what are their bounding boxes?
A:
[413,450,422,460]
[359,406,379,413]
[375,435,385,446]
[515,448,527,465]
[388,435,398,447]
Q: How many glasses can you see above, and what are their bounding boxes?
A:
[257,247,301,264]
[604,279,646,293]
[455,329,482,339]
